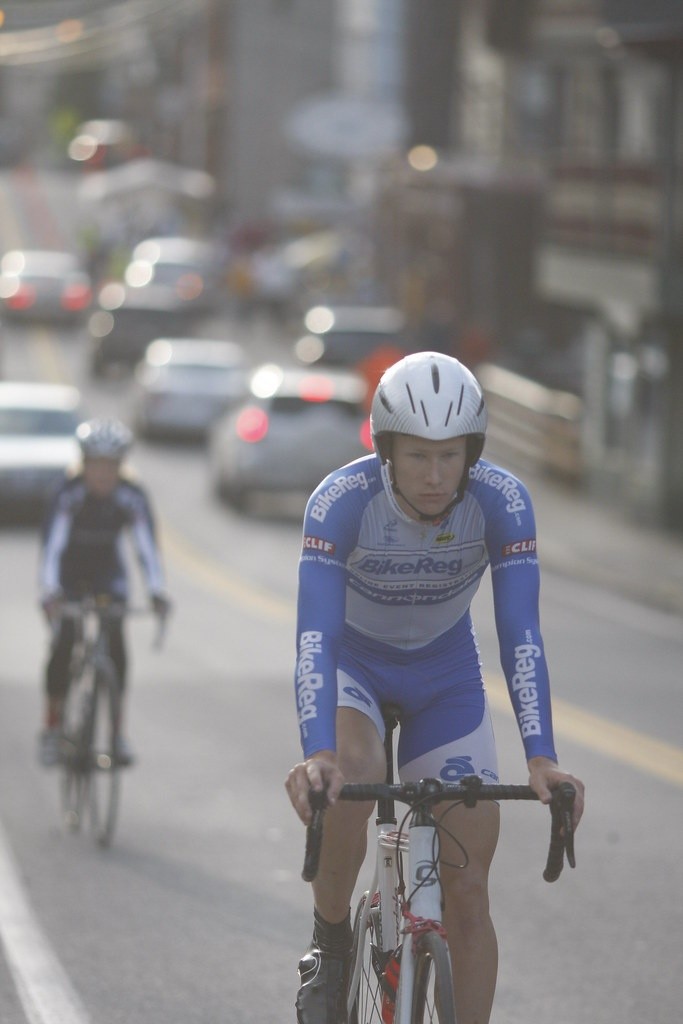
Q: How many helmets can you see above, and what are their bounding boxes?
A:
[370,351,489,466]
[76,416,134,457]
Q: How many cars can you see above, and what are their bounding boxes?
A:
[124,336,252,451]
[0,114,227,376]
[209,367,380,514]
[0,383,88,519]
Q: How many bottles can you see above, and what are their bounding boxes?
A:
[381,945,402,1024]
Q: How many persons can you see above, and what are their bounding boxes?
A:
[285,352,586,1023]
[34,417,172,768]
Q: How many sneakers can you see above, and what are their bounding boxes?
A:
[113,737,135,765]
[295,939,354,1024]
[40,730,58,766]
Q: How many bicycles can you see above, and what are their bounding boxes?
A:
[36,588,168,850]
[293,775,576,1024]
